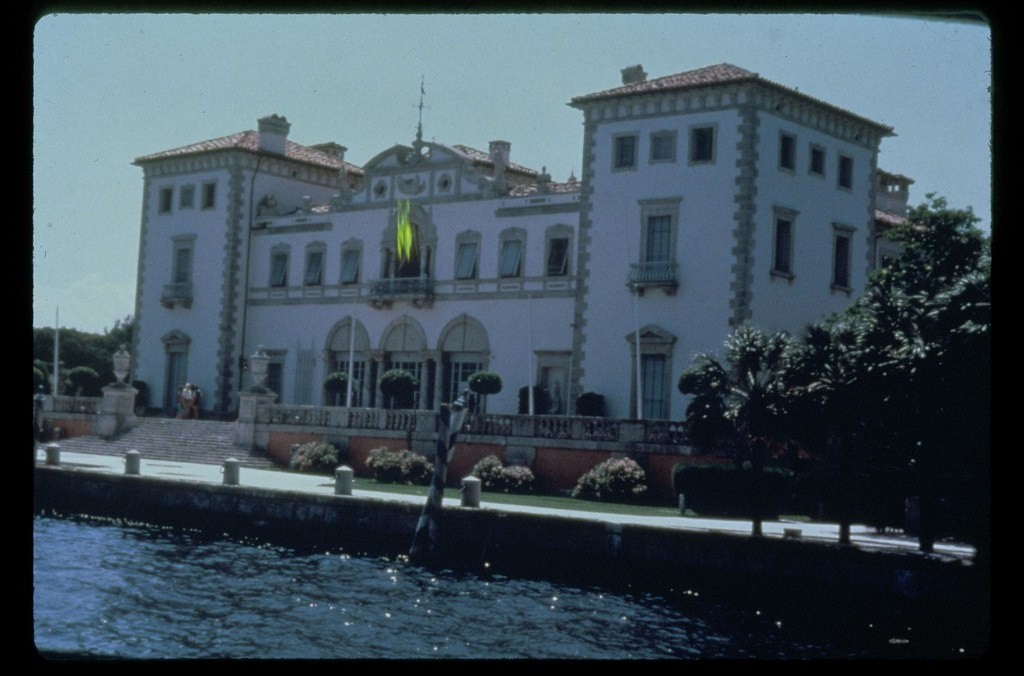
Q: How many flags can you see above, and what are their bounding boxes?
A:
[396,197,412,260]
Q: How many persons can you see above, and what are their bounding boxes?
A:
[176,383,204,420]
